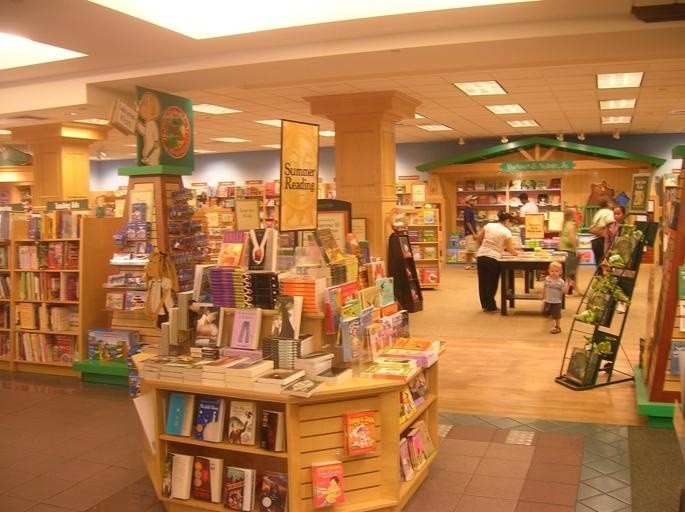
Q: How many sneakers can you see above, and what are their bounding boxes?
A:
[616,305,626,313]
[491,308,501,313]
[464,264,476,270]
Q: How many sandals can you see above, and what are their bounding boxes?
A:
[549,327,561,333]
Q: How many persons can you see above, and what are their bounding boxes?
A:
[137,92,161,165]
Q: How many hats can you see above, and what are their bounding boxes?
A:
[465,194,479,203]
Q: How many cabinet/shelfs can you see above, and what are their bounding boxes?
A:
[0,179,329,387]
[448,174,562,271]
[386,233,426,313]
[129,344,449,510]
[394,205,445,292]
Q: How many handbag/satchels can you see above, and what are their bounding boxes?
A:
[587,218,605,235]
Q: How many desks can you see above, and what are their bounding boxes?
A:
[494,247,568,314]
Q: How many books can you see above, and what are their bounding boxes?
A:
[0,178,684,512]
